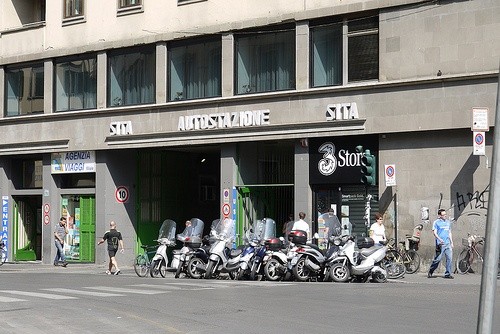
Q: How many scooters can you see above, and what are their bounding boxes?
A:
[205,218,249,279]
[184,235,238,279]
[250,228,285,280]
[175,218,204,278]
[237,227,255,279]
[264,239,300,280]
[328,232,388,283]
[150,219,180,278]
[288,230,355,280]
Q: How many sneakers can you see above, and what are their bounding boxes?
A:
[114,269,120,275]
[105,271,111,275]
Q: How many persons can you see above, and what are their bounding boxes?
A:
[427,209,455,279]
[283,213,294,241]
[369,214,387,244]
[318,209,342,251]
[177,221,195,246]
[54,217,69,267]
[98,221,124,275]
[292,211,310,240]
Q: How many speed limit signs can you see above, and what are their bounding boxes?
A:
[115,186,129,203]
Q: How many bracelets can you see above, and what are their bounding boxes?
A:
[121,248,124,249]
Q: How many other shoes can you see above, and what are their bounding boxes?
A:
[54,263,59,266]
[62,262,68,267]
[445,275,454,279]
[428,272,432,277]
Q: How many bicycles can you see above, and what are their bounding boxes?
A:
[456,233,500,277]
[133,244,166,278]
[0,241,7,266]
[381,234,420,279]
